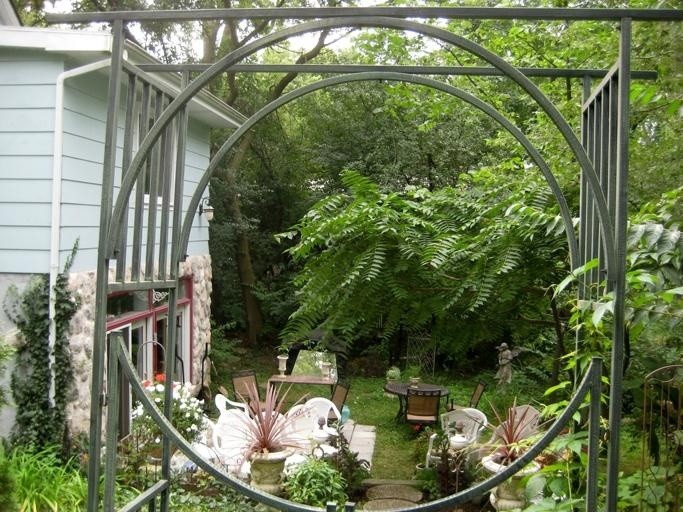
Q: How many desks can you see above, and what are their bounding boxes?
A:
[382,382,450,423]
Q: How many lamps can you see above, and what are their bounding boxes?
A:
[196,195,214,221]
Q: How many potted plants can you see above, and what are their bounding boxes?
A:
[407,365,421,389]
[230,377,312,496]
[473,394,543,510]
[273,345,288,379]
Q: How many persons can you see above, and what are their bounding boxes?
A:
[495,343,513,384]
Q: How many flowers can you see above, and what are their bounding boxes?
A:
[132,372,207,444]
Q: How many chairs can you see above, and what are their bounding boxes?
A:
[402,378,486,468]
[214,367,350,460]
